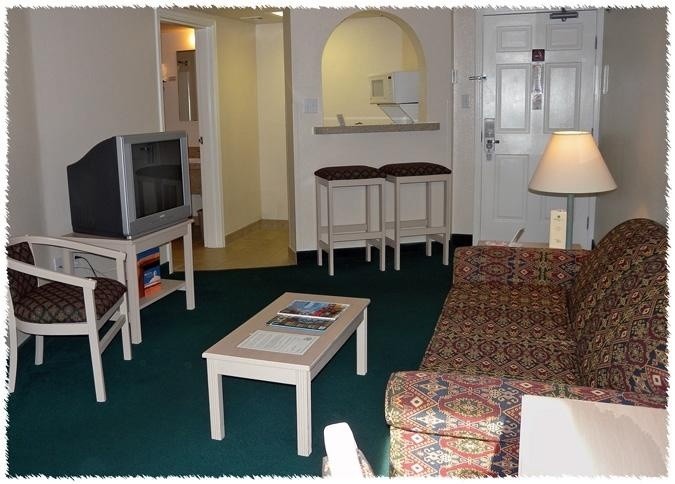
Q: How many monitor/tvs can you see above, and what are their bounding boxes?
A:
[67,130,191,240]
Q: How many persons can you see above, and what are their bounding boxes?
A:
[149,270,161,283]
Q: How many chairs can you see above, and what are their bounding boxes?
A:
[4,234,133,403]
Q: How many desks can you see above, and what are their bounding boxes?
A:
[516,390,667,478]
[63,219,197,345]
[202,293,370,457]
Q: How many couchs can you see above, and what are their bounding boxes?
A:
[383,216,668,476]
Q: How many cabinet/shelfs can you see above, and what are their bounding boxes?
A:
[187,147,202,197]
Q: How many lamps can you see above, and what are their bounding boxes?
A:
[528,130,617,249]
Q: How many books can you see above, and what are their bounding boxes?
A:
[265,312,340,331]
[277,298,350,320]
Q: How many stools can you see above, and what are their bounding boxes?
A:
[312,160,451,277]
